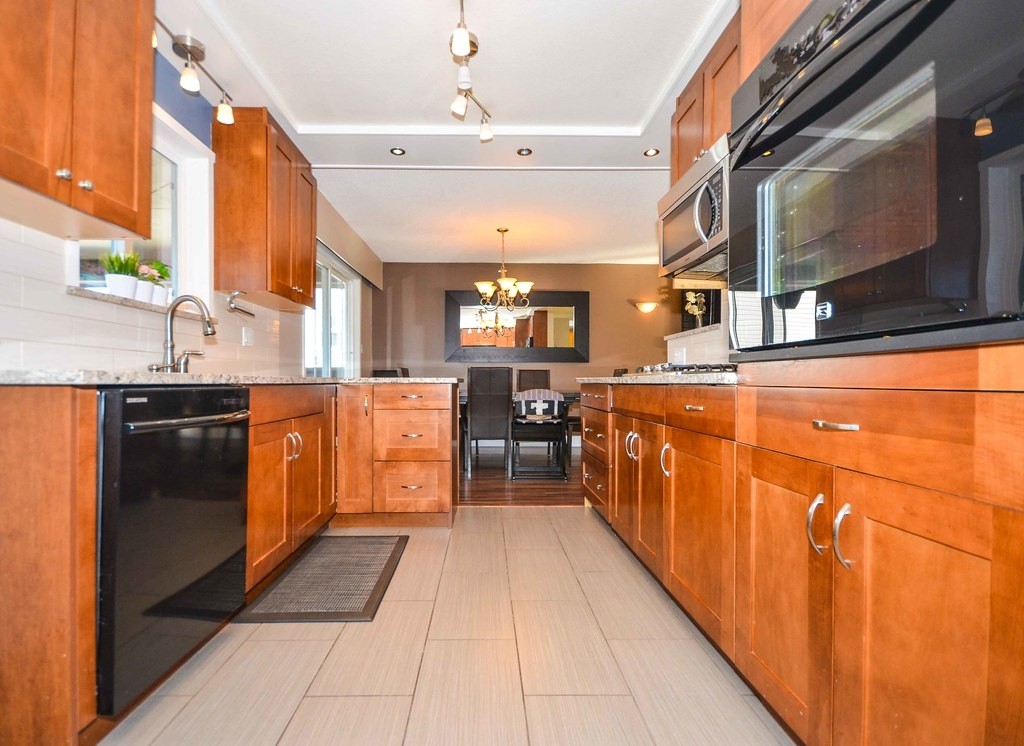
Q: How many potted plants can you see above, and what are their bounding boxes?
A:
[152,260,170,306]
[99,250,139,299]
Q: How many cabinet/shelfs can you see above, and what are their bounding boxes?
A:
[611,383,665,588]
[0,0,156,240]
[666,384,736,663]
[249,383,453,590]
[670,5,742,188]
[580,383,611,520]
[212,105,318,312]
[738,341,1024,746]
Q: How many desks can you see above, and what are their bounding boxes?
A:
[459,388,580,470]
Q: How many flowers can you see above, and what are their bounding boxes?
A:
[136,264,158,280]
[685,291,706,314]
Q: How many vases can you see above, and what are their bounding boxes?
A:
[696,314,703,328]
[135,280,154,303]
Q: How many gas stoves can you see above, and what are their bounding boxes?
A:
[620,364,739,386]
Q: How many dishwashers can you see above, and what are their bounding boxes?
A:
[99,386,250,716]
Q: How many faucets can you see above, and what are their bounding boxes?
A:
[162,295,216,372]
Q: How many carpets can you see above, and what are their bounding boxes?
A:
[227,535,410,621]
[140,544,245,623]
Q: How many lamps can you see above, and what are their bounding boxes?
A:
[657,277,673,305]
[477,307,514,337]
[447,1,498,143]
[636,302,659,313]
[215,90,234,125]
[172,36,207,91]
[474,228,535,311]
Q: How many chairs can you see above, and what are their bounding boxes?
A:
[568,368,628,468]
[512,387,567,481]
[397,367,409,377]
[466,366,513,482]
[516,369,551,392]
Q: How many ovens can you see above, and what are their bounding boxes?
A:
[727,0,1024,362]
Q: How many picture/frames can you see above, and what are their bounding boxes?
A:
[445,289,590,363]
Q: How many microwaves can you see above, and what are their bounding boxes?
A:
[657,132,731,281]
